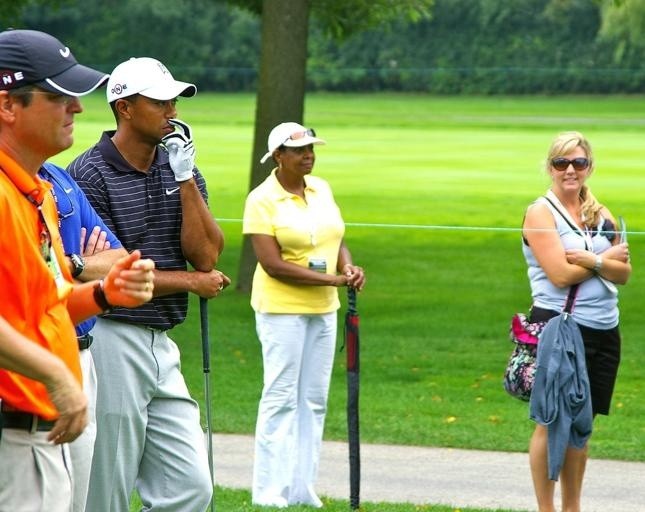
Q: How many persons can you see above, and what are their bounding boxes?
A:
[0,29,155,512]
[521,129,633,512]
[37,161,130,512]
[242,121,366,509]
[64,55,231,512]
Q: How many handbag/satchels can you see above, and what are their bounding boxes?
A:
[504,313,549,403]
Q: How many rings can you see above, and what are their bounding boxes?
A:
[219,285,224,291]
[144,282,149,291]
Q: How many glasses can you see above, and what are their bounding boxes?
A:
[279,127,317,143]
[549,158,591,172]
[59,207,75,222]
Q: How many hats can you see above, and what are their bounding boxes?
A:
[107,56,197,101]
[1,27,111,97]
[259,122,325,162]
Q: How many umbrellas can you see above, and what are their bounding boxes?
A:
[340,286,362,510]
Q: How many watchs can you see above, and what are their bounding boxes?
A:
[66,251,85,279]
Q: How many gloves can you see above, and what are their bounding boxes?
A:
[160,119,195,182]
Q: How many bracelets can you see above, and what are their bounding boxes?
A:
[594,254,601,273]
[93,280,113,315]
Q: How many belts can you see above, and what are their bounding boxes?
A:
[77,334,93,352]
[2,411,56,432]
